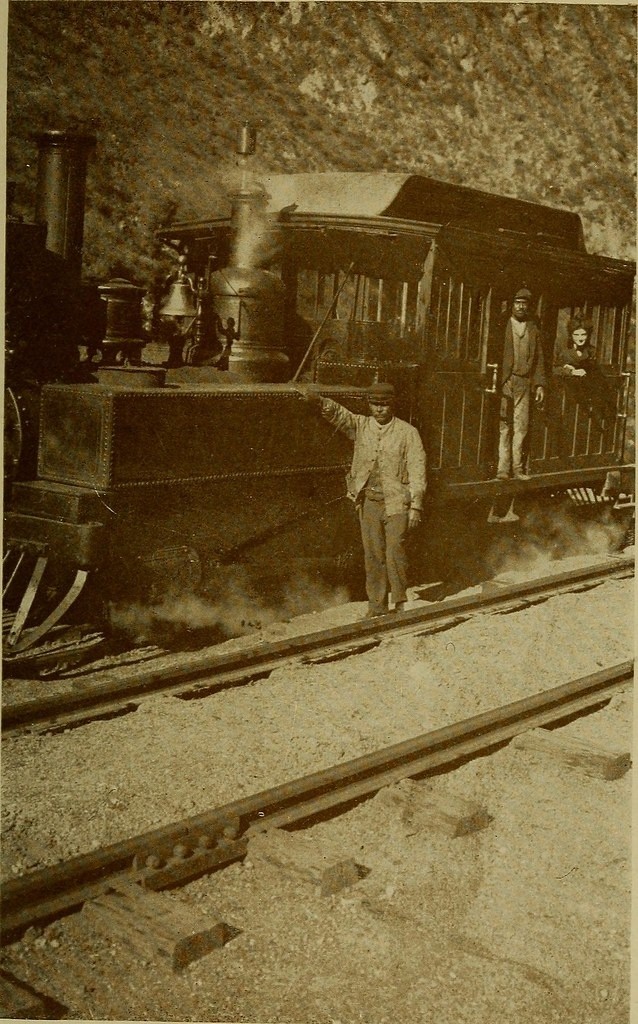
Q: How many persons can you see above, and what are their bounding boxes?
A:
[293,382,428,619]
[497,288,599,481]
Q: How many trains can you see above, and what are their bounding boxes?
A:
[4,119,636,661]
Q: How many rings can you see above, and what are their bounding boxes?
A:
[419,520,421,522]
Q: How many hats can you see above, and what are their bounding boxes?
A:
[368,382,395,405]
[508,288,532,300]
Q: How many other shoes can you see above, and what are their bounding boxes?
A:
[395,602,404,613]
[357,609,386,623]
[514,474,531,480]
[496,473,508,479]
